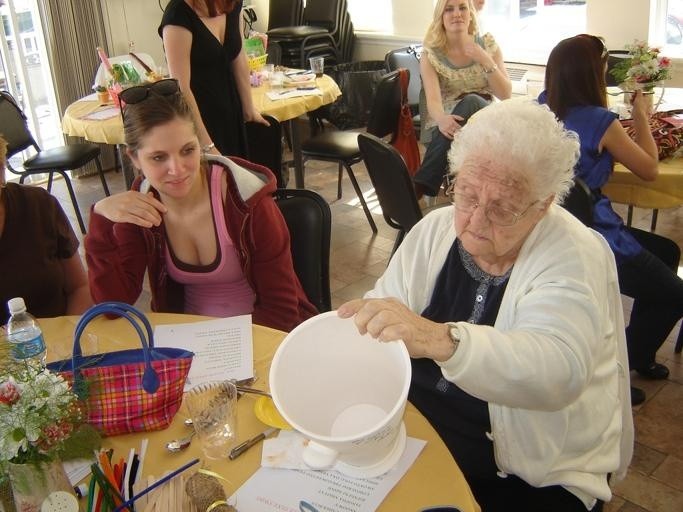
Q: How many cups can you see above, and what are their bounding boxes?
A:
[186,382,239,458]
[309,56,324,78]
[267,70,283,91]
[269,312,413,482]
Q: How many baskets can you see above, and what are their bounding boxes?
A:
[249,54,268,68]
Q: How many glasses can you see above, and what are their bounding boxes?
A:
[445,177,542,227]
[117,79,188,123]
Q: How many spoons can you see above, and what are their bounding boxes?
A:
[165,377,256,453]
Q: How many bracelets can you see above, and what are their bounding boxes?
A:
[482,64,496,74]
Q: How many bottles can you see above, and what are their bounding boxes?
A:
[6,296,47,367]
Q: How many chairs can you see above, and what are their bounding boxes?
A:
[560,177,644,405]
[599,51,658,231]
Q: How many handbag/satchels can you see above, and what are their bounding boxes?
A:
[44,302,195,437]
[393,104,420,176]
[626,112,683,161]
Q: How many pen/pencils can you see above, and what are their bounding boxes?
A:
[278,90,295,95]
[229,427,279,460]
[88,439,199,511]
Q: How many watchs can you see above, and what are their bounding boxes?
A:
[200,142,214,153]
[447,323,460,358]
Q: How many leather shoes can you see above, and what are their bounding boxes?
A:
[631,386,646,405]
[637,363,669,380]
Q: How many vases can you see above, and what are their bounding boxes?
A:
[623,82,655,115]
[5,459,82,511]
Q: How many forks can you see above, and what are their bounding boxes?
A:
[607,91,654,98]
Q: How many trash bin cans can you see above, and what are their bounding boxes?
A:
[333,60,388,127]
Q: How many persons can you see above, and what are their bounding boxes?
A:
[412,1,515,212]
[156,1,289,192]
[0,137,93,322]
[538,34,682,379]
[83,78,325,336]
[337,99,636,512]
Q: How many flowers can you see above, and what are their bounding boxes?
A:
[0,342,105,504]
[611,37,670,88]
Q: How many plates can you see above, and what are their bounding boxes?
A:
[253,393,293,431]
